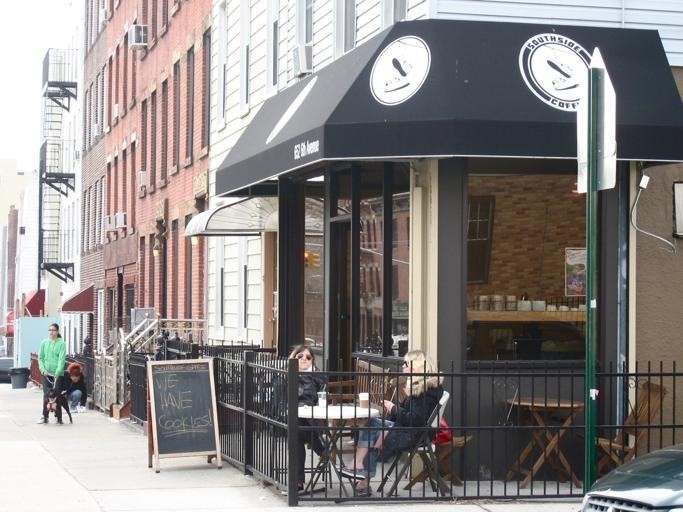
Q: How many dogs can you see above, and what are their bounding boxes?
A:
[42,389,74,425]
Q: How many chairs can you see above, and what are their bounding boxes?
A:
[324,359,391,464]
[379,390,450,497]
[403,437,471,495]
[595,381,668,478]
[271,380,334,489]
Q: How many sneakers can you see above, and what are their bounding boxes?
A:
[70,407,78,413]
[337,467,366,479]
[353,482,372,496]
[37,417,49,424]
[281,483,304,496]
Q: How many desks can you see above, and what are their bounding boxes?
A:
[505,398,584,490]
[281,405,378,504]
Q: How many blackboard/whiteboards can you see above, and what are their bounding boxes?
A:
[146,358,221,458]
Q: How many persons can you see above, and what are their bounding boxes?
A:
[37,328,67,424]
[58,366,88,415]
[335,349,444,498]
[268,347,332,470]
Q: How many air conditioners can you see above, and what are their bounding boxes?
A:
[128,24,149,50]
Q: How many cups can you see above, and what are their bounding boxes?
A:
[359,392,370,409]
[317,392,330,410]
[517,300,588,311]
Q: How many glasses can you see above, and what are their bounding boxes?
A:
[296,353,312,360]
[400,360,409,367]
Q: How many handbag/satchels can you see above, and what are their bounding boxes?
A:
[432,417,451,444]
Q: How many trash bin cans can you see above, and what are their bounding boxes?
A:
[9,367,28,388]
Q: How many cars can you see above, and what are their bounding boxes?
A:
[579,442,683,512]
